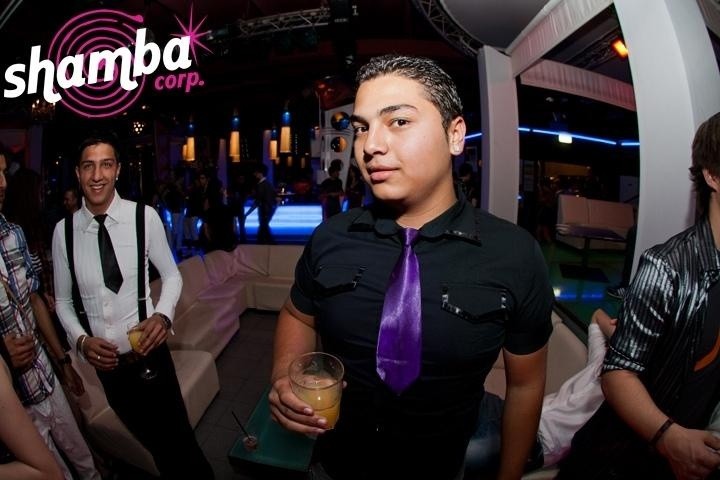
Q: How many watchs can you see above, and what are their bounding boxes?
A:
[56,355,73,364]
[154,311,175,329]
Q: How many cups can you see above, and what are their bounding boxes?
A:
[12,323,32,338]
[288,352,345,430]
[126,321,145,355]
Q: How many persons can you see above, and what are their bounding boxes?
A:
[52,135,219,478]
[145,164,278,250]
[0,355,62,478]
[344,166,366,208]
[465,309,624,480]
[318,164,344,219]
[456,162,479,203]
[62,187,81,215]
[265,52,555,479]
[0,155,101,480]
[605,110,719,480]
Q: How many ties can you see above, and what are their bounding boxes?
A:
[92,213,124,296]
[374,227,428,393]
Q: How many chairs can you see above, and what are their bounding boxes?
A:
[63,244,305,476]
[556,194,638,250]
[483,310,588,480]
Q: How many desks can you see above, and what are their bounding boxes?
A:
[553,224,626,283]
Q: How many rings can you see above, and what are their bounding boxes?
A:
[95,354,101,363]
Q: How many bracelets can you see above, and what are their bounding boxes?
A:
[646,416,678,452]
[79,335,86,358]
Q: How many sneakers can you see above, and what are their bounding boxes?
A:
[607,286,626,299]
